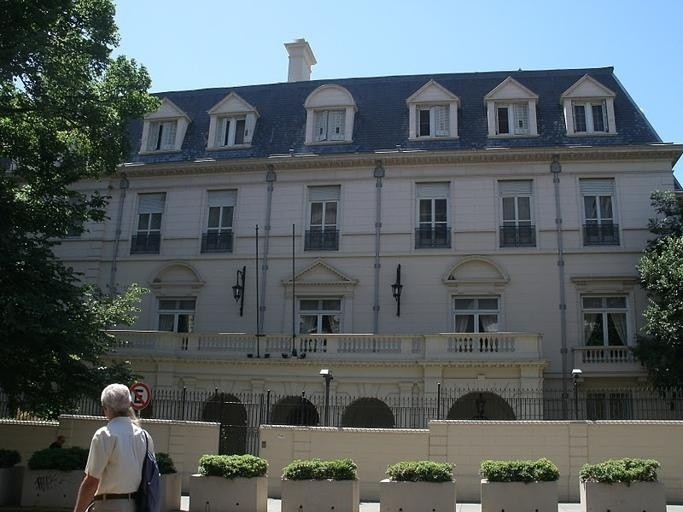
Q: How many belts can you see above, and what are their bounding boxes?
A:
[94,492,138,500]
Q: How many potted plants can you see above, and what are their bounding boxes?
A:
[479,456,560,511]
[579,457,663,512]
[189,454,270,512]
[154,451,182,512]
[1,445,95,512]
[281,456,361,511]
[378,461,457,512]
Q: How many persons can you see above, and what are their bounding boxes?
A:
[71,382,159,510]
[51,435,65,449]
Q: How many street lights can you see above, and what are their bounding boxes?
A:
[572,368,582,420]
[320,369,333,427]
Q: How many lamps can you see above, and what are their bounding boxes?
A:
[390,262,404,317]
[232,263,247,317]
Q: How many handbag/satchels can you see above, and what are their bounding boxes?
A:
[135,431,162,511]
